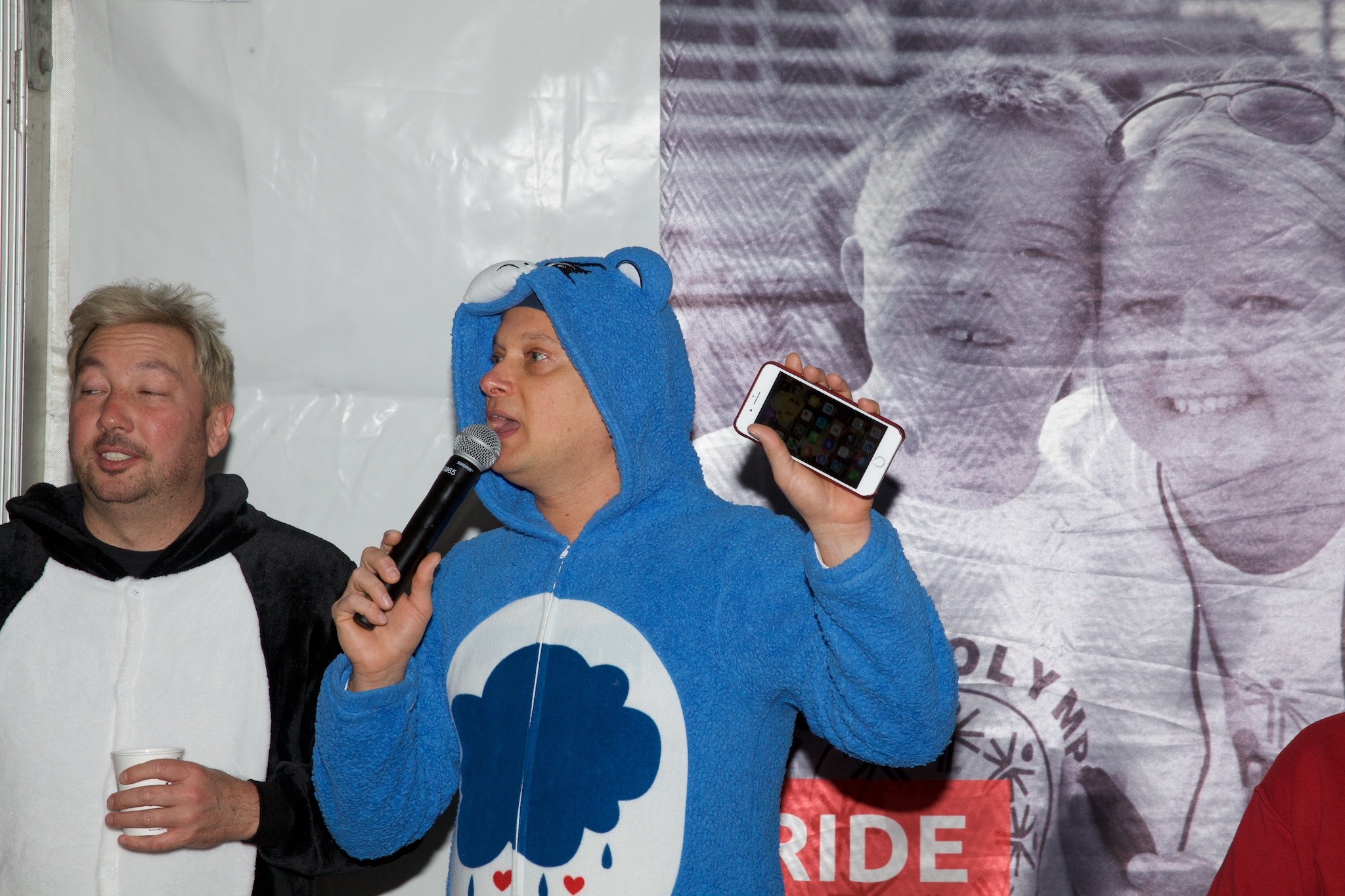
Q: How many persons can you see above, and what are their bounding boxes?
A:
[690,42,1251,896]
[1036,64,1345,805]
[311,247,962,896]
[0,285,460,896]
[1207,708,1345,896]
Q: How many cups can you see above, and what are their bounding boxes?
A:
[115,750,182,838]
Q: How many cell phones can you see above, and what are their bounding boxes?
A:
[733,361,906,500]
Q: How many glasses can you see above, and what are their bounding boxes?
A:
[1105,79,1345,169]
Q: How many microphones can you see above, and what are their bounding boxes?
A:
[352,424,502,633]
